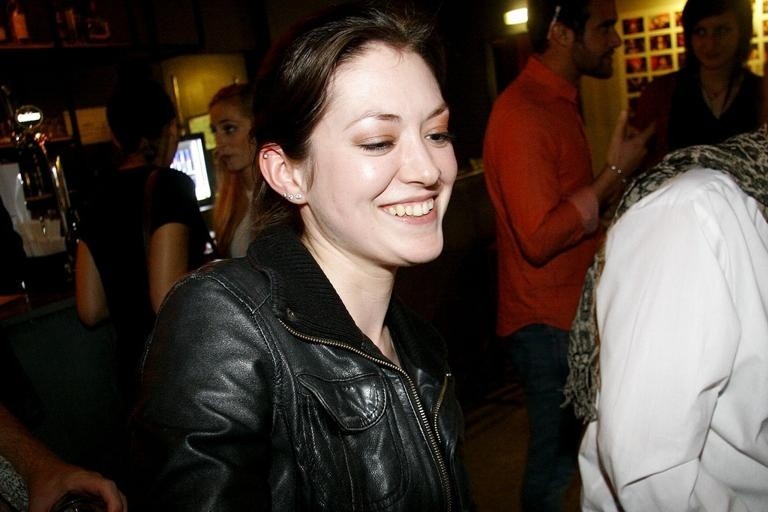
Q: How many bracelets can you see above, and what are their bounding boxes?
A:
[607,162,624,177]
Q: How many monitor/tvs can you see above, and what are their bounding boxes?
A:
[170,132,215,207]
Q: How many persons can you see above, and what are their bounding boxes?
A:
[209,84,260,260]
[483,1,655,511]
[629,1,768,183]
[118,6,477,510]
[560,74,768,511]
[1,405,128,510]
[75,85,208,367]
